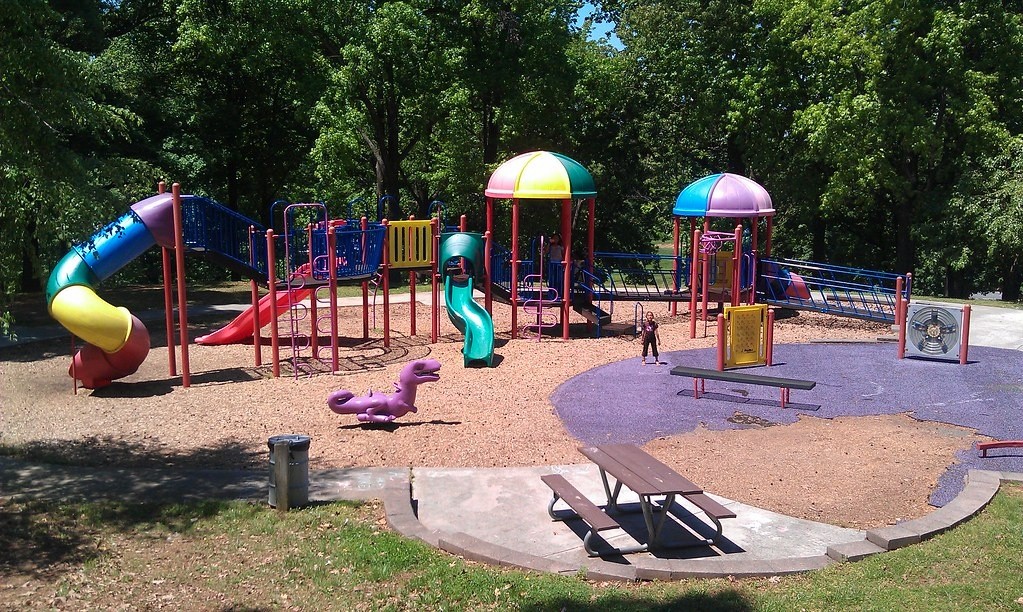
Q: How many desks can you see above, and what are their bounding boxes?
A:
[547,443,723,556]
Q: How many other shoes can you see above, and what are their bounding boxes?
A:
[642,359,645,366]
[655,360,660,366]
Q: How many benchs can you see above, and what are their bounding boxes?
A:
[669,366,817,408]
[679,491,738,519]
[540,474,621,531]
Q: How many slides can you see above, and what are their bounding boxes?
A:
[762,266,813,304]
[195,260,323,345]
[44,191,176,391]
[445,273,495,365]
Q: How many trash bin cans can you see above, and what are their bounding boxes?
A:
[268,434,310,508]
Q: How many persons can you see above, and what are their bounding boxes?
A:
[545,234,564,261]
[641,311,661,366]
